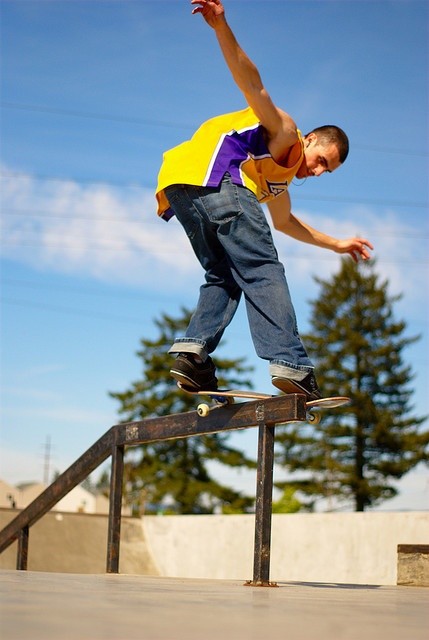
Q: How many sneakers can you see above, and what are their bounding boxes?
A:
[169,353,218,390]
[272,367,323,400]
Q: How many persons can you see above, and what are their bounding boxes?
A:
[155,0,374,400]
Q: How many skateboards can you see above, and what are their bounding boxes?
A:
[177,380,350,424]
